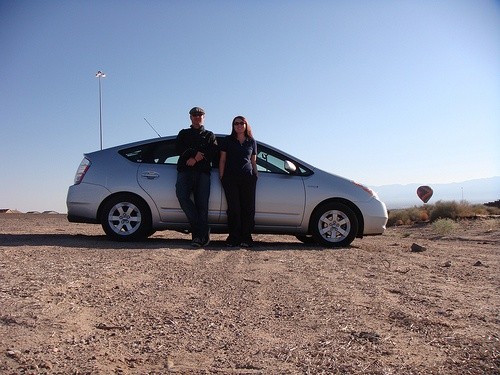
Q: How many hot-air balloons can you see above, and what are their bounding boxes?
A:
[416,185,434,206]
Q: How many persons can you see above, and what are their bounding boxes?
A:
[176,106,219,247]
[219,116,258,248]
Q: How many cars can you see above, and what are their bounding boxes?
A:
[65,117,388,248]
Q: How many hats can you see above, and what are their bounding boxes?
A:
[189,107,205,115]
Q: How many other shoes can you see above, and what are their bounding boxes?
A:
[224,241,240,247]
[240,240,250,247]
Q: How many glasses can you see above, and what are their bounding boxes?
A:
[234,122,246,125]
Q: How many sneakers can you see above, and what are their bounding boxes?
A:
[203,227,210,246]
[191,236,201,247]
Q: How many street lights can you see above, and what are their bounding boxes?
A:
[94,70,106,150]
